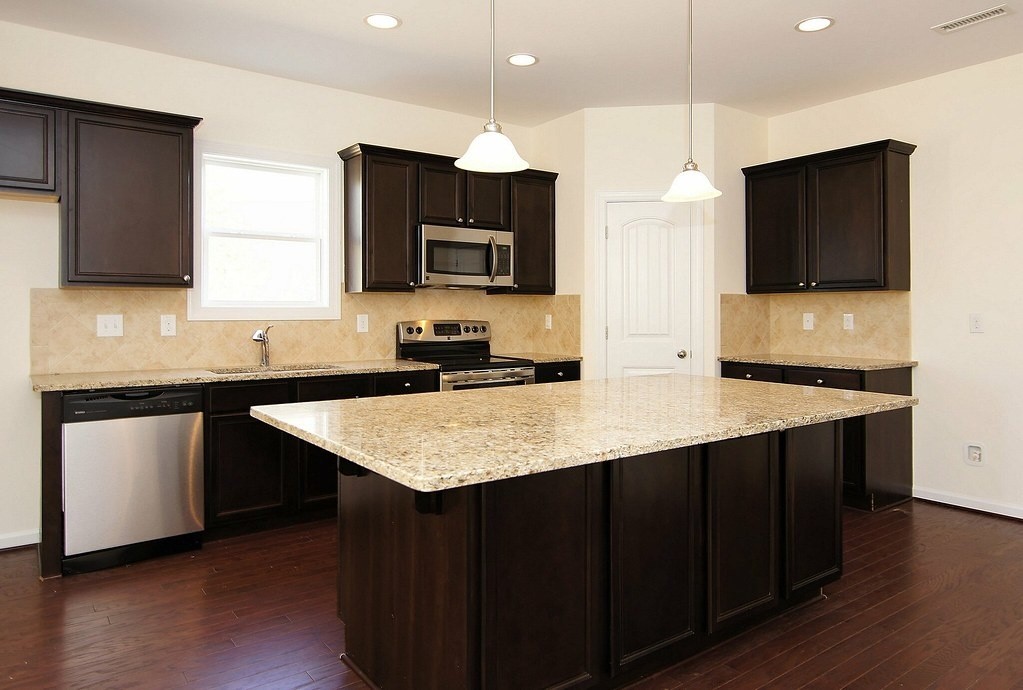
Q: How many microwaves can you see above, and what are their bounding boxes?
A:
[414,223,515,290]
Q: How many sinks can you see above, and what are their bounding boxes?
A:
[206,363,344,374]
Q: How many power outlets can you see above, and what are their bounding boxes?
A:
[545,315,552,329]
[844,314,853,329]
[161,314,176,337]
[357,314,369,333]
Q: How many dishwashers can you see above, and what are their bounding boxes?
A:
[61,386,205,558]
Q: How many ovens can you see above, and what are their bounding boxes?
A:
[441,366,535,391]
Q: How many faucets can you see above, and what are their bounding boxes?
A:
[252,325,274,366]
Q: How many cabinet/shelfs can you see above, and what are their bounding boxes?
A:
[719,362,914,511]
[0,86,64,197]
[420,151,511,232]
[203,379,300,540]
[742,140,918,292]
[515,167,559,296]
[358,142,416,292]
[301,370,439,522]
[63,97,203,288]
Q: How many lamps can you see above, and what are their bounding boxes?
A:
[660,2,722,202]
[455,0,529,172]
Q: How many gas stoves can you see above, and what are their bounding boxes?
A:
[397,319,534,371]
